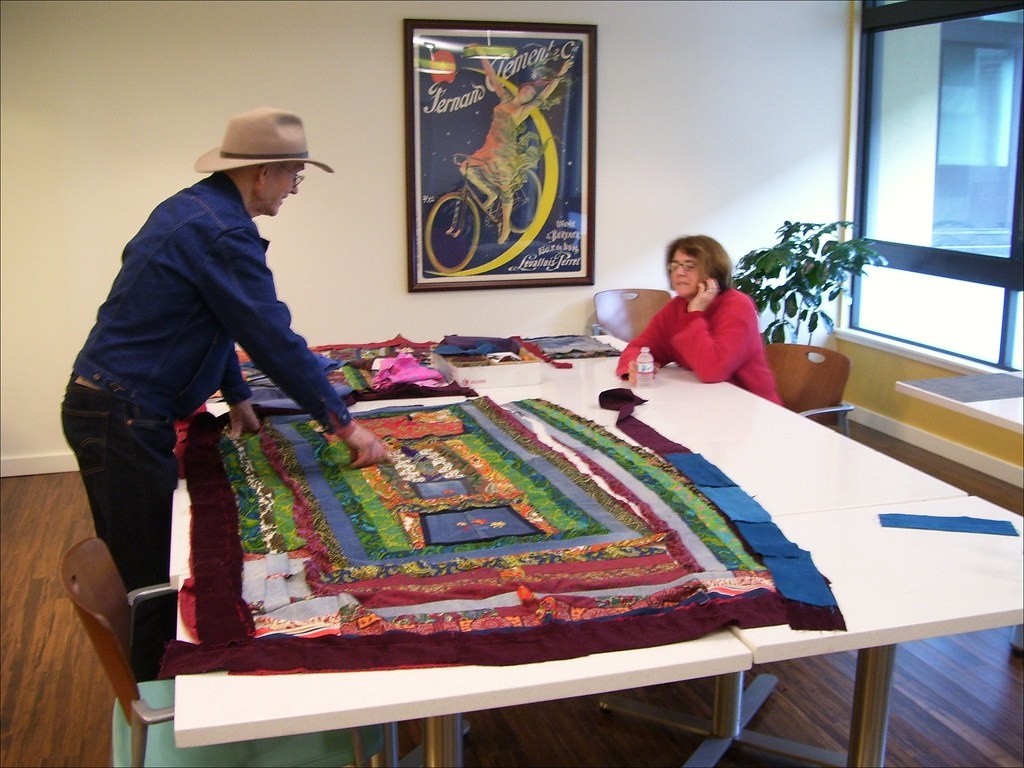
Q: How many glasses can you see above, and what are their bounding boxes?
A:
[278,161,304,187]
[667,259,702,271]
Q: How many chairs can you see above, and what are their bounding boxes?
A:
[590,290,674,343]
[762,343,855,437]
[60,538,385,768]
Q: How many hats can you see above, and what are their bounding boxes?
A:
[193,108,335,174]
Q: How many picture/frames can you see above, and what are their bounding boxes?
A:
[403,18,598,292]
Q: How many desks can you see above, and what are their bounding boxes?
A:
[172,336,1023,768]
[895,372,1024,435]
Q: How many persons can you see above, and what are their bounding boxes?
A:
[616,236,783,406]
[62,109,393,682]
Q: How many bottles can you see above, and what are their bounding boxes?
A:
[636,346,655,390]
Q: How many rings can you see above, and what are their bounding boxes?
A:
[706,289,711,292]
